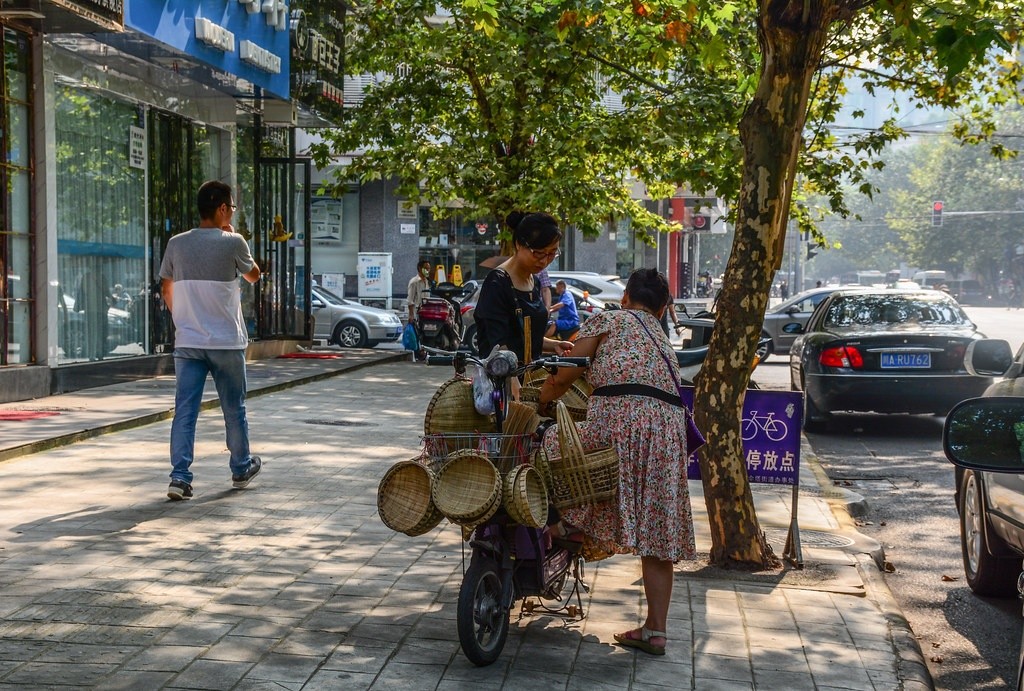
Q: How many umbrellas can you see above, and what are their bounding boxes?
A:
[479,256,510,269]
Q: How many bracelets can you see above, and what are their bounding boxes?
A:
[533,394,546,405]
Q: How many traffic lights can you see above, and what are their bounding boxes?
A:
[932,200,944,226]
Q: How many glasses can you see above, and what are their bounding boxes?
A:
[217,200,236,212]
[521,235,561,259]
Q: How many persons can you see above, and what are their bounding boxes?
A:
[533,268,697,654]
[534,269,580,337]
[780,278,788,302]
[1006,276,1020,310]
[473,210,574,387]
[407,260,431,361]
[73,259,168,361]
[810,281,826,311]
[159,181,262,499]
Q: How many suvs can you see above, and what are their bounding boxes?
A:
[458,271,630,357]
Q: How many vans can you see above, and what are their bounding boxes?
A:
[914,270,949,291]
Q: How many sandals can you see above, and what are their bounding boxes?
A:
[614,625,667,655]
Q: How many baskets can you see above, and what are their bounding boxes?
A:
[377,367,621,538]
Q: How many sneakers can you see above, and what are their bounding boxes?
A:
[167,480,194,499]
[232,456,263,488]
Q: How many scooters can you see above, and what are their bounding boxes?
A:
[671,288,773,389]
[415,281,472,355]
[425,352,594,669]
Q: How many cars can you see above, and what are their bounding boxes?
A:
[753,282,996,433]
[6,273,133,359]
[294,285,403,348]
[953,341,1024,599]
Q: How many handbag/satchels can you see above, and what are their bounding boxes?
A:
[685,412,706,458]
[402,319,419,350]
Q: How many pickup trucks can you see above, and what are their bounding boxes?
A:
[954,278,984,305]
